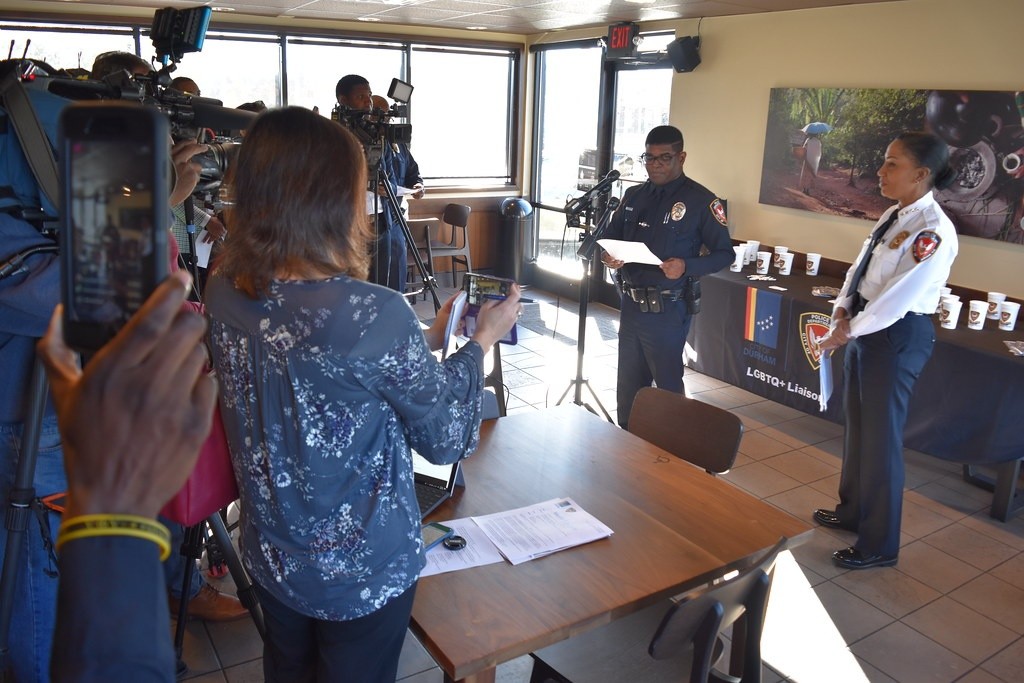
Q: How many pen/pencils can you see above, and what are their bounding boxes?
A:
[483,294,537,302]
[663,212,670,224]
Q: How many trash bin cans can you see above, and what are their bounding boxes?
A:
[494,197,535,286]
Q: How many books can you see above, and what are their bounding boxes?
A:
[442,291,467,362]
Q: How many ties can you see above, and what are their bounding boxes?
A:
[845,207,904,299]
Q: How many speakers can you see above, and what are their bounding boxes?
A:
[667,36,701,73]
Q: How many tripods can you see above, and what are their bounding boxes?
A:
[555,204,616,427]
[358,149,442,320]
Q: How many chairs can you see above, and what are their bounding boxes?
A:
[627,386,744,476]
[417,203,472,287]
[403,218,439,304]
[525,536,786,683]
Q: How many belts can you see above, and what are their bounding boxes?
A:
[620,281,686,300]
[858,294,928,316]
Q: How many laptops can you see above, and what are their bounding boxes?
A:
[411,447,460,521]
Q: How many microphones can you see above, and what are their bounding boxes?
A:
[564,170,621,261]
[163,102,261,131]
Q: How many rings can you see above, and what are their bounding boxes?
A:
[517,312,521,317]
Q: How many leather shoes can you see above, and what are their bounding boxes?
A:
[831,544,899,568]
[813,508,856,531]
[168,583,250,622]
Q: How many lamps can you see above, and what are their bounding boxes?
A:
[596,23,645,58]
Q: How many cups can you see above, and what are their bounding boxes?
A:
[773,246,789,268]
[940,300,963,330]
[998,302,1021,331]
[968,300,990,330]
[729,246,746,273]
[806,253,821,276]
[739,243,752,266]
[939,294,960,321]
[756,252,772,275]
[779,253,794,276]
[746,240,760,262]
[987,292,1007,320]
[939,287,952,296]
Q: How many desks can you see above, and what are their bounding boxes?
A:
[682,238,1024,522]
[406,401,814,683]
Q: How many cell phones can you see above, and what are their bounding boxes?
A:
[58,101,170,357]
[422,520,455,551]
[463,273,514,306]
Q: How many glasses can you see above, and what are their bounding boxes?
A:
[640,152,681,167]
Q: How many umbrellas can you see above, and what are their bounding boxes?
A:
[801,123,834,138]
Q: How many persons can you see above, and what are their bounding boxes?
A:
[139,210,154,257]
[813,132,958,569]
[1,50,522,681]
[801,135,821,194]
[599,126,735,431]
[102,216,120,260]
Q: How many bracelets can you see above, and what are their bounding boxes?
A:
[56,514,171,561]
